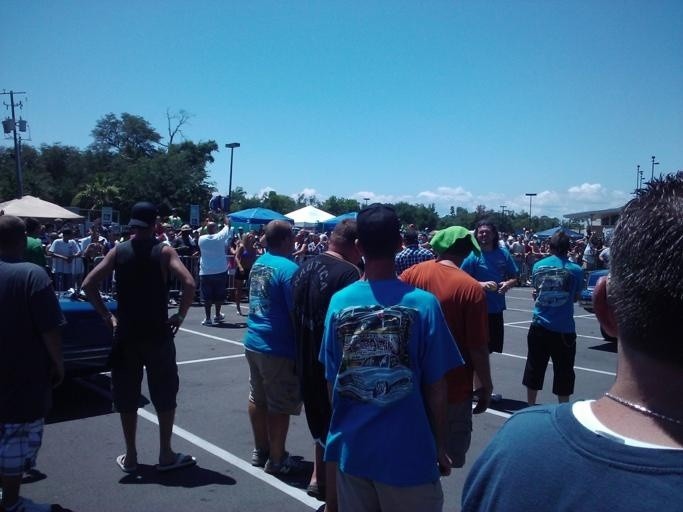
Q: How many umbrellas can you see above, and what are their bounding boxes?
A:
[322,211,358,225]
[226,206,295,225]
[0,195,89,219]
[285,205,336,224]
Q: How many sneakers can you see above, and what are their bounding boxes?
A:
[214,314,226,323]
[471,386,503,403]
[202,319,213,326]
[0,494,64,512]
[306,484,327,503]
[250,447,312,478]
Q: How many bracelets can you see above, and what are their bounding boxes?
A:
[175,309,186,321]
[102,314,113,319]
[477,363,491,383]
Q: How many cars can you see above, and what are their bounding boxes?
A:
[581,267,616,344]
[42,294,118,391]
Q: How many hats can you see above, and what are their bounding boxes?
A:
[298,228,329,241]
[180,223,192,232]
[127,200,156,228]
[356,202,401,250]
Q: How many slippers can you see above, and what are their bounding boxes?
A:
[115,453,139,473]
[155,452,199,472]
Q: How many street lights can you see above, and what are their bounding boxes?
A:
[224,142,241,212]
[651,156,659,182]
[500,204,509,235]
[636,164,645,197]
[524,193,536,226]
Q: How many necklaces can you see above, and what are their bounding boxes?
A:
[600,391,682,428]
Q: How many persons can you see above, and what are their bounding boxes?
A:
[243,218,314,476]
[395,228,439,275]
[0,215,68,512]
[198,208,225,326]
[156,216,267,305]
[521,233,586,405]
[235,232,257,315]
[398,225,494,469]
[25,216,131,296]
[460,174,681,510]
[289,218,362,501]
[80,200,198,473]
[460,218,519,403]
[318,201,470,512]
[500,233,611,287]
[292,227,329,264]
